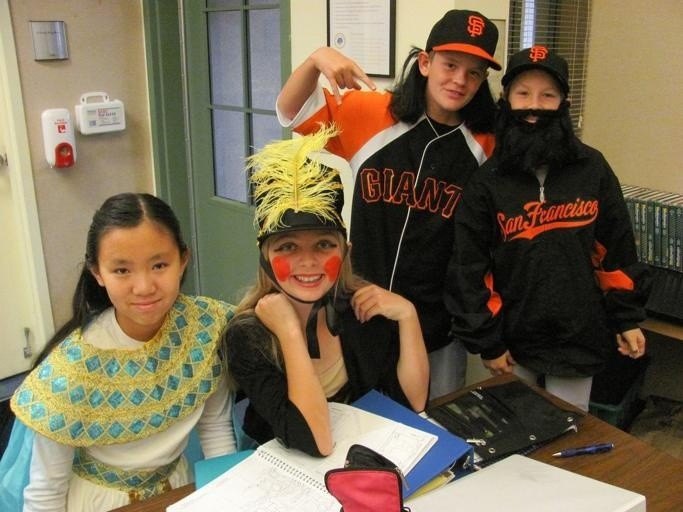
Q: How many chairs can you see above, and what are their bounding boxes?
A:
[233,388,260,451]
[0,369,33,462]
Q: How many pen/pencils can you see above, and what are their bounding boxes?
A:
[551,443,616,460]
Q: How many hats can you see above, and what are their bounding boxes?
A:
[500,49,570,90]
[426,10,503,71]
[253,158,345,232]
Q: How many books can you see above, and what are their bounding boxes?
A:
[615,185,681,275]
[165,389,472,509]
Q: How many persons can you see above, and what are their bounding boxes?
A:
[456,46,648,414]
[224,139,434,460]
[269,8,499,406]
[1,191,257,511]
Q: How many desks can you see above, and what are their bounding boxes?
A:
[104,371,683,511]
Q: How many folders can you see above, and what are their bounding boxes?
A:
[336,388,474,504]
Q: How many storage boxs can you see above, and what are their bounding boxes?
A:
[536,347,649,430]
